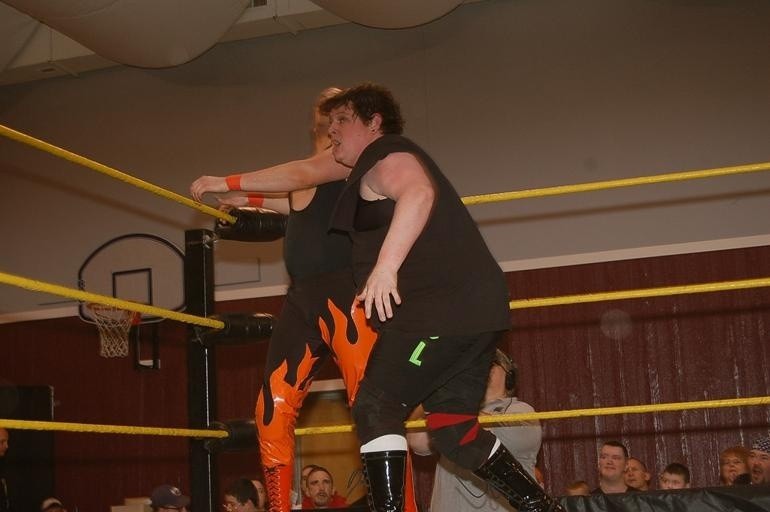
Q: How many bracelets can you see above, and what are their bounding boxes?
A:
[246,193,265,208]
[225,174,242,191]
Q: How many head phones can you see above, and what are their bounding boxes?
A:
[494,362,516,389]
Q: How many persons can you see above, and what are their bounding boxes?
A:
[0,428,15,512]
[534,434,770,495]
[301,464,347,505]
[190,83,418,512]
[252,479,266,508]
[317,81,570,512]
[302,467,345,508]
[150,485,191,512]
[406,334,543,512]
[41,497,67,512]
[223,479,260,512]
